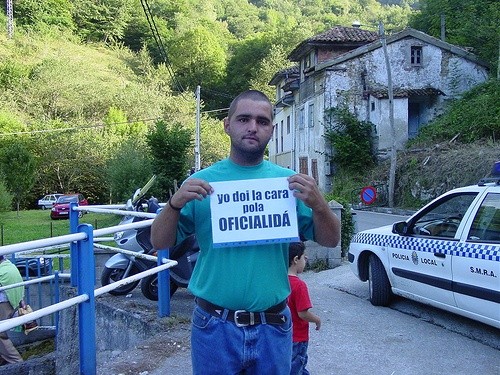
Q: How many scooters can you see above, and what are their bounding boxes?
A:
[99,191,201,300]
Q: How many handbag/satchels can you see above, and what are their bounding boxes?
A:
[18,305,38,335]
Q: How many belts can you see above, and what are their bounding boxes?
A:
[196,297,288,327]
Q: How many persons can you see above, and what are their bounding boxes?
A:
[0,255,25,363]
[288,242,322,375]
[150,90,340,375]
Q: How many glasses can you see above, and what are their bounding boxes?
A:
[298,256,308,261]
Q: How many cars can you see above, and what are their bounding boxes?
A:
[346,161,500,328]
[6,254,54,278]
[50,193,88,219]
[37,193,63,210]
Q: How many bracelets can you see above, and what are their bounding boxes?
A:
[169,197,183,212]
